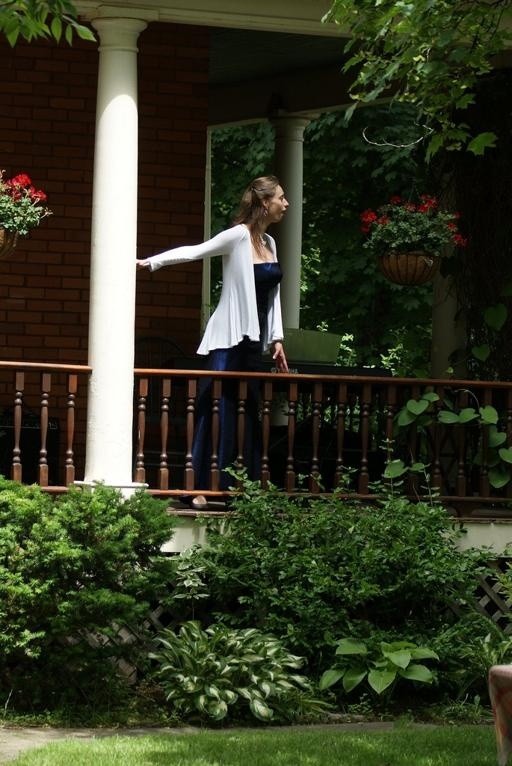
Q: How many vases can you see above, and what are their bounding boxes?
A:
[379,249,442,287]
[0,229,21,261]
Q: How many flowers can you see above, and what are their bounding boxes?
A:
[0,168,54,237]
[360,193,470,260]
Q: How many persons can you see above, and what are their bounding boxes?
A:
[136,176,293,511]
